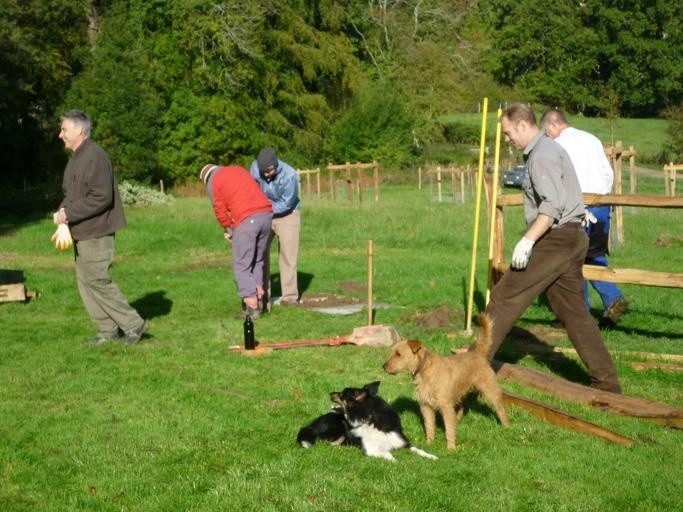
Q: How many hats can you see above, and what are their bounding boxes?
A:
[200,162,218,187]
[257,147,279,173]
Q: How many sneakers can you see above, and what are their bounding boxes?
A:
[598,296,630,329]
[240,295,298,320]
[549,316,568,333]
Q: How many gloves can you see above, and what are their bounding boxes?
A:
[580,210,598,228]
[510,237,535,270]
[51,223,73,250]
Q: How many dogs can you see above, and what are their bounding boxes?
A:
[381,310,511,454]
[295,380,440,463]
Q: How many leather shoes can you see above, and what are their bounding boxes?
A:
[83,332,121,347]
[124,320,151,347]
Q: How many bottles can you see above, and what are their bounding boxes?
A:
[243,314,256,350]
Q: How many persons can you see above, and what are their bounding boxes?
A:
[541,109,628,328]
[467,106,621,395]
[250,148,302,306]
[53,111,146,344]
[199,164,273,319]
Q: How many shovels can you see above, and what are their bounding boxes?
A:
[228,324,401,351]
